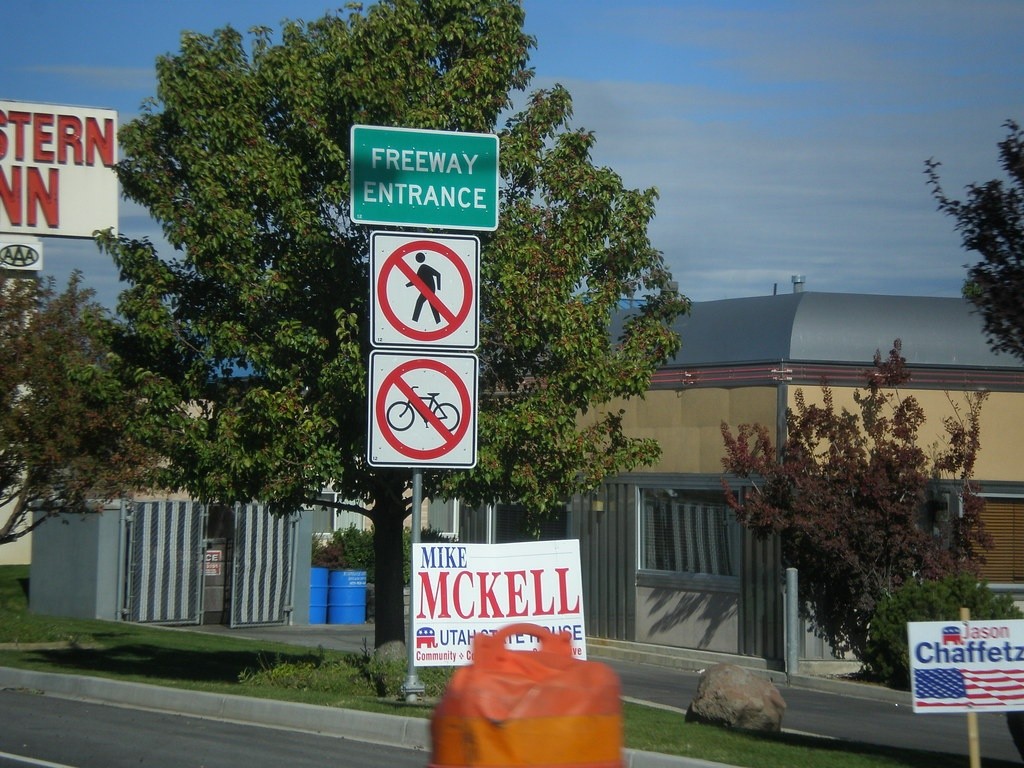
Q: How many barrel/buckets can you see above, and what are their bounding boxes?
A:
[328,570,367,624]
[309,567,329,626]
